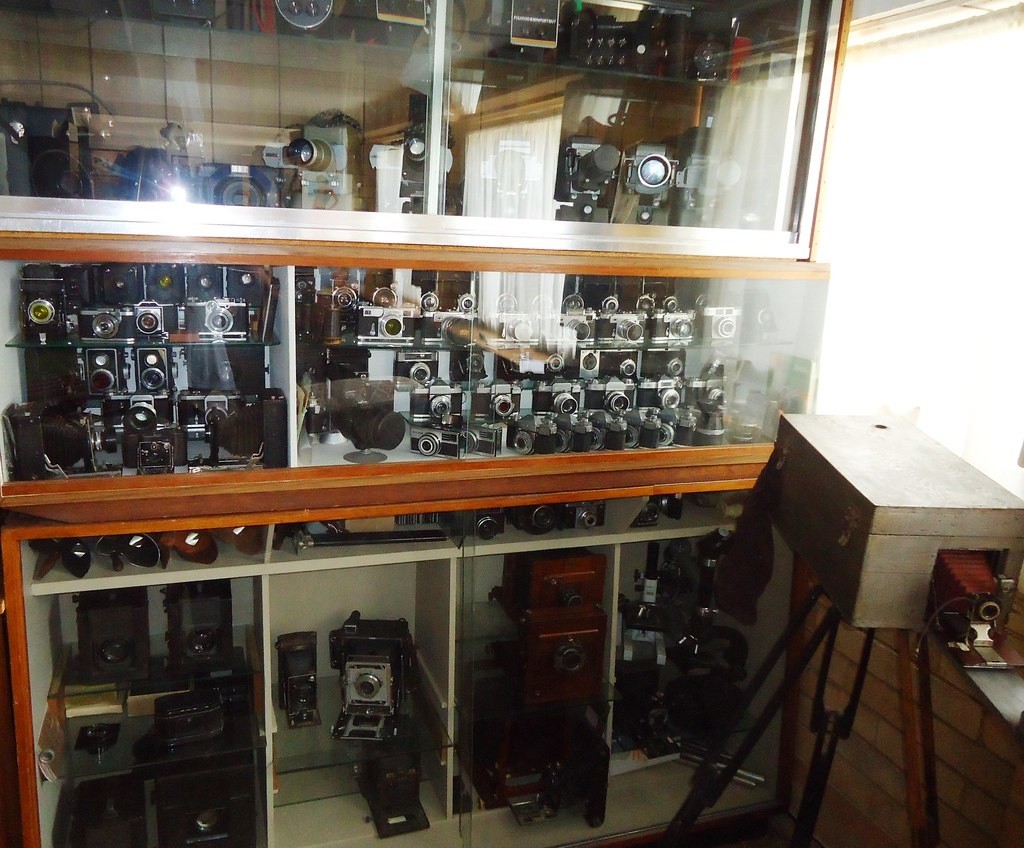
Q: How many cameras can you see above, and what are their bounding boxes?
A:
[0,0,747,848]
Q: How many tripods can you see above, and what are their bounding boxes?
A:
[655,585,941,848]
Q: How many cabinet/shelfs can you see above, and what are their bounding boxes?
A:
[0,0,858,507]
[0,478,807,848]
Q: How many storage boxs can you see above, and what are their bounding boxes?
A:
[776,411,1024,629]
